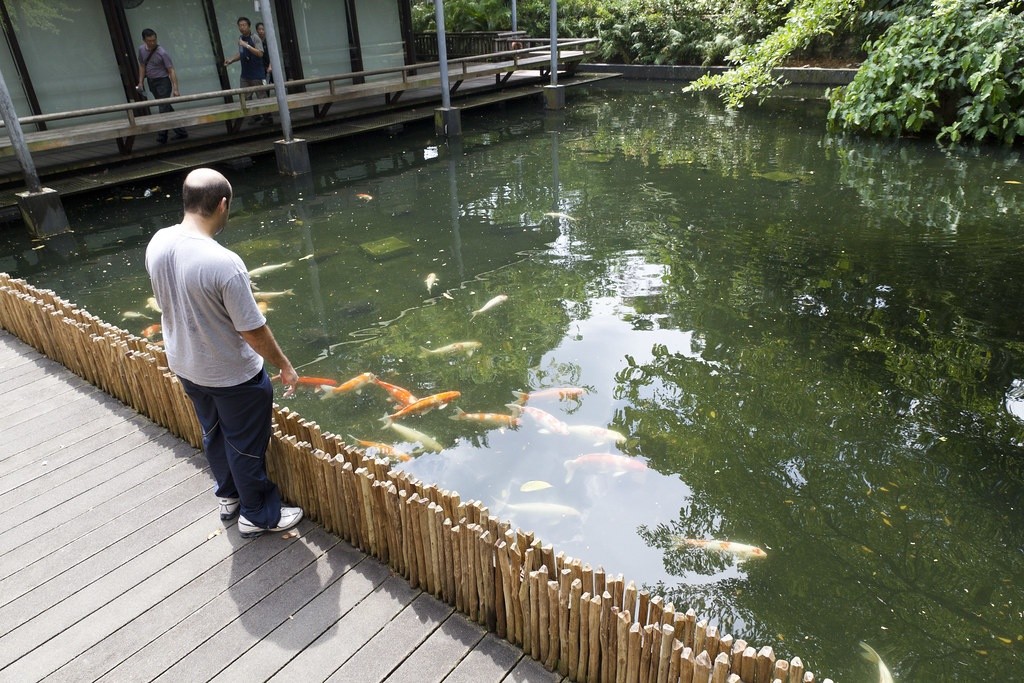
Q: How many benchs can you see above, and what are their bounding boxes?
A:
[0,36,598,164]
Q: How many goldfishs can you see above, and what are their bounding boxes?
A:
[352,193,373,199]
[855,640,897,683]
[123,252,645,520]
[663,534,768,562]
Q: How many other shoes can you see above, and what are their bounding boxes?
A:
[260,118,273,126]
[248,117,262,125]
[170,133,188,140]
[156,138,167,144]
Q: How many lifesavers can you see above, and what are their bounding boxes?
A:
[511,41,523,59]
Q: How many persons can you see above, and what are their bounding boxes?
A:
[223,17,273,132]
[248,21,278,125]
[143,168,305,538]
[137,28,189,143]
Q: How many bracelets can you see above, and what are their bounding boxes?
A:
[247,45,250,49]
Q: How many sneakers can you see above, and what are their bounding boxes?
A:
[238,507,303,538]
[219,496,240,520]
